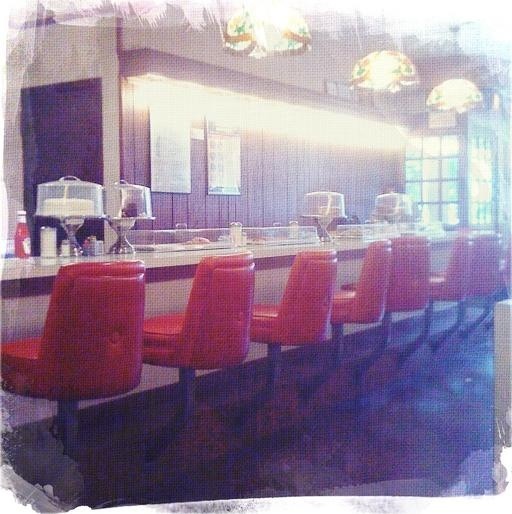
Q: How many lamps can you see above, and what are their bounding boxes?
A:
[221,3,483,115]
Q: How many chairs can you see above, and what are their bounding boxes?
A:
[397,235,473,366]
[349,236,435,392]
[297,238,396,418]
[436,239,501,353]
[1,256,150,473]
[138,249,259,431]
[223,246,343,427]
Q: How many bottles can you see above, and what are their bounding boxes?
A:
[14,210,32,260]
[61,240,71,258]
